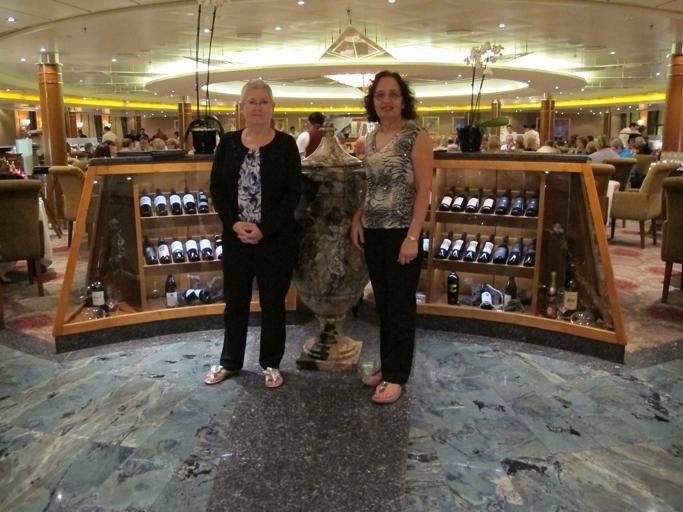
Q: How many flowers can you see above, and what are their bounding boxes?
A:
[462,39,512,127]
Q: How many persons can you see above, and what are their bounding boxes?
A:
[523,125,529,133]
[628,126,647,145]
[586,141,597,155]
[136,128,150,142]
[576,135,589,154]
[133,136,153,151]
[611,138,632,157]
[77,129,85,136]
[203,80,303,387]
[630,136,652,154]
[94,144,111,158]
[350,70,434,403]
[526,125,541,150]
[119,139,134,151]
[288,126,300,139]
[151,127,168,140]
[505,125,517,140]
[588,135,621,163]
[295,111,327,158]
[169,133,182,146]
[153,139,167,151]
[501,135,517,150]
[102,141,116,156]
[445,138,457,149]
[101,126,117,142]
[620,123,639,145]
[165,139,182,150]
[77,142,94,159]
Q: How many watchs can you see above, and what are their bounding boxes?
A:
[404,233,419,242]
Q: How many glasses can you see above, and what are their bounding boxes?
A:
[242,98,273,107]
[373,89,403,99]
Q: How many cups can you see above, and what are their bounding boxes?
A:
[570,310,594,327]
[93,308,105,320]
[107,298,118,317]
[80,307,96,319]
[555,289,570,322]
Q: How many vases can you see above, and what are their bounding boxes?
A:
[455,123,485,151]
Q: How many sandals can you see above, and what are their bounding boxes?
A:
[362,369,384,387]
[371,379,403,406]
[261,365,286,389]
[203,361,242,385]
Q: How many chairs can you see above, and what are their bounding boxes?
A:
[633,155,659,175]
[608,158,683,249]
[658,151,680,160]
[48,165,86,249]
[582,162,617,224]
[602,156,641,228]
[1,176,45,298]
[659,176,683,306]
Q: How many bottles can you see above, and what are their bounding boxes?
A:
[563,264,577,320]
[452,186,469,212]
[465,186,482,213]
[481,284,493,310]
[165,273,178,308]
[153,187,169,216]
[197,188,209,213]
[435,230,454,260]
[199,235,215,261]
[85,266,108,317]
[511,185,525,216]
[215,234,226,260]
[142,232,158,265]
[447,271,458,305]
[157,234,172,264]
[169,187,183,215]
[183,186,197,214]
[449,231,466,260]
[492,235,509,264]
[183,288,208,301]
[170,232,185,262]
[477,234,494,262]
[504,273,517,312]
[139,188,154,217]
[524,237,537,266]
[186,230,199,262]
[463,232,480,262]
[438,186,456,210]
[421,230,429,256]
[495,186,510,215]
[525,187,541,216]
[546,271,558,319]
[508,237,523,265]
[480,184,497,214]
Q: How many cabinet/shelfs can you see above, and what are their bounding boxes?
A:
[52,158,636,347]
